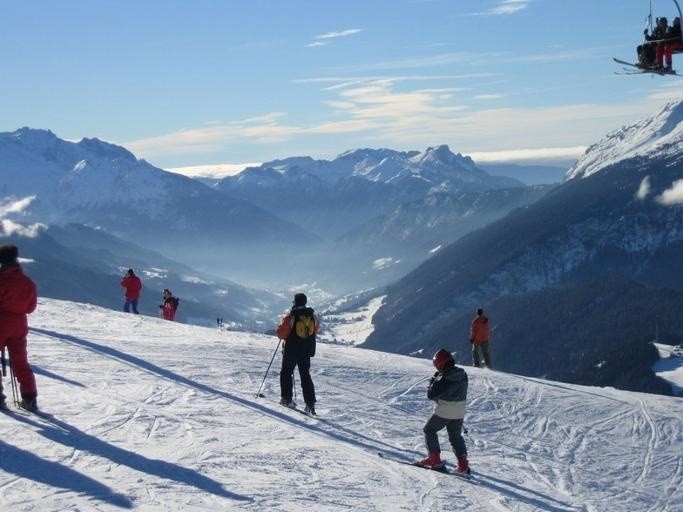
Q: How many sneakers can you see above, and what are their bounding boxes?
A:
[421,450,442,466]
[453,457,470,476]
[279,397,298,408]
[19,396,39,412]
[303,404,319,418]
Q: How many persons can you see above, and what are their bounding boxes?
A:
[119,268,142,315]
[410,347,471,474]
[468,306,492,370]
[651,17,682,73]
[275,292,320,416]
[158,288,179,321]
[636,17,672,66]
[0,242,39,413]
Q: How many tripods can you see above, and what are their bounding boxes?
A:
[254,336,299,401]
[0,344,20,411]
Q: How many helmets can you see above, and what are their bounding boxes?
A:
[433,350,456,369]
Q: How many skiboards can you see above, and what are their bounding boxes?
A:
[2,400,53,421]
[380,452,485,486]
[612,56,679,77]
[264,392,324,421]
